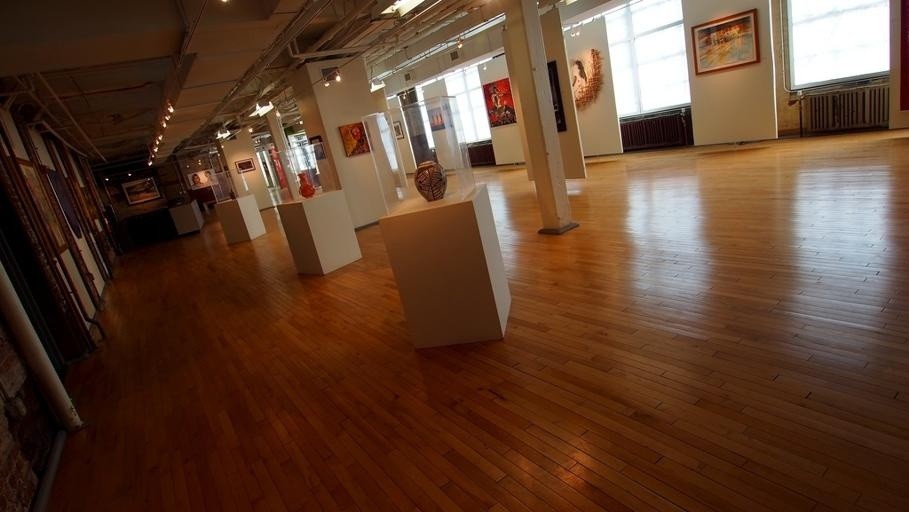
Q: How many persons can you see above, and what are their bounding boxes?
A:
[204,171,215,185]
[191,174,204,188]
[349,125,369,157]
[490,86,506,114]
[572,61,587,100]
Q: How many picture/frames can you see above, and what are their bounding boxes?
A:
[235,158,256,174]
[690,8,760,76]
[15,157,70,255]
[121,173,164,207]
[310,136,326,160]
[392,121,404,140]
[547,62,567,133]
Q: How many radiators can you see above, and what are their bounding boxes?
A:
[802,83,890,133]
[620,107,694,154]
